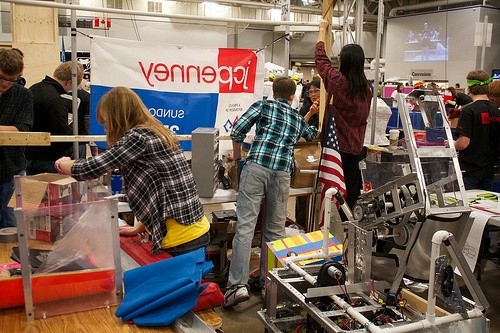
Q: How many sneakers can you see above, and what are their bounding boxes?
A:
[221,285,249,308]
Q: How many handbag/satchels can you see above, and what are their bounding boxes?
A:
[115,246,214,326]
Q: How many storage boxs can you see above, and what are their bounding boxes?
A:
[7,172,80,242]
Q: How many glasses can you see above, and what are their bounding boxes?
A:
[0,76,19,83]
[308,89,320,94]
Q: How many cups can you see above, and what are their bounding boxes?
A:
[389,129,400,149]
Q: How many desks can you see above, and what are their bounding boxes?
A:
[104,178,325,272]
[366,144,458,187]
[0,219,223,333]
[429,188,500,281]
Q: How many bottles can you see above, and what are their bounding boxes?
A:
[111,168,124,195]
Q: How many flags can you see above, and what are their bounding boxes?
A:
[315,104,347,226]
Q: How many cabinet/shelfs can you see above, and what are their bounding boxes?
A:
[191,127,220,199]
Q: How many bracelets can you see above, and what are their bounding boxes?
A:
[56,156,71,173]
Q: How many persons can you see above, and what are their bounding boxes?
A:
[0,61,500,308]
[408,22,445,61]
[315,18,372,221]
